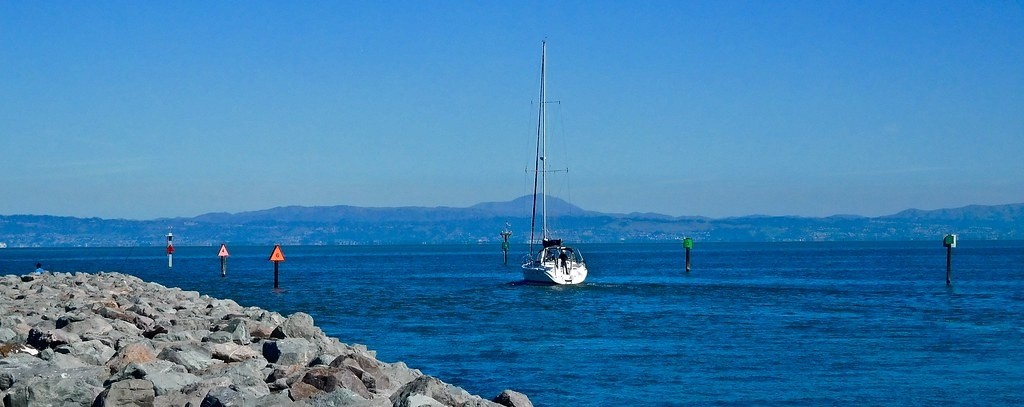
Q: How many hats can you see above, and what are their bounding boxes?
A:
[562,250,566,252]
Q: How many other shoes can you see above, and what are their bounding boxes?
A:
[566,273,568,274]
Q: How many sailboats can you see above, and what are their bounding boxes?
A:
[518,37,589,284]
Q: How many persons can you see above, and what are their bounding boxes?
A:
[559,252,569,274]
[34,262,45,274]
